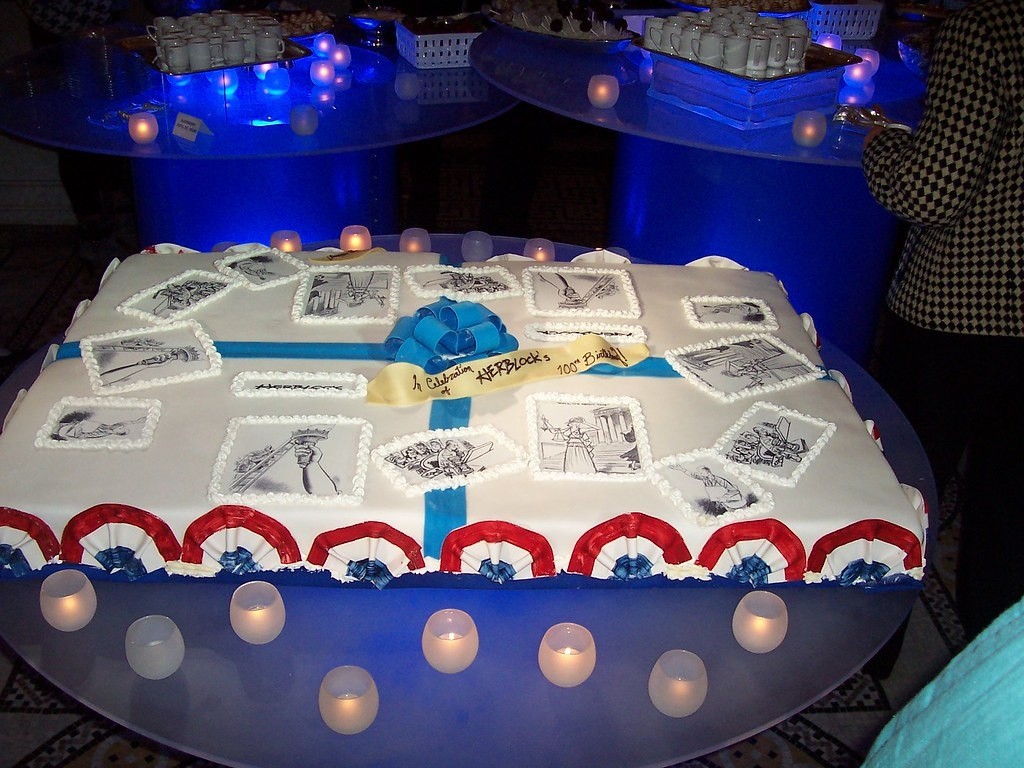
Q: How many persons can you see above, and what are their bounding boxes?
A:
[367,0,552,241]
[17,0,193,271]
[860,0,1024,680]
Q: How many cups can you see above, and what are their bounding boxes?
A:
[815,33,879,88]
[648,650,708,718]
[792,110,826,146]
[145,9,352,95]
[461,231,494,263]
[396,101,422,124]
[170,63,353,135]
[586,107,619,125]
[732,591,789,654]
[134,143,161,157]
[394,73,421,100]
[643,4,814,78]
[125,615,184,680]
[538,622,596,688]
[319,665,379,735]
[340,225,372,252]
[129,113,158,144]
[399,228,432,252]
[523,237,556,261]
[605,247,631,260]
[39,569,97,632]
[588,75,619,109]
[421,608,479,674]
[838,81,876,106]
[270,230,302,253]
[230,581,286,644]
[212,241,238,252]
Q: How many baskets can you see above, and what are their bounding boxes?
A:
[394,17,484,70]
[805,1,883,39]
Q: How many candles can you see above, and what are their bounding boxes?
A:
[559,647,579,655]
[439,632,462,639]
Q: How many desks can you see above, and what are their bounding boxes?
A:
[470,9,946,362]
[0,233,940,767]
[0,23,522,251]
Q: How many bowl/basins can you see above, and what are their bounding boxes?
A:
[480,2,641,56]
[898,36,933,78]
[348,13,407,46]
